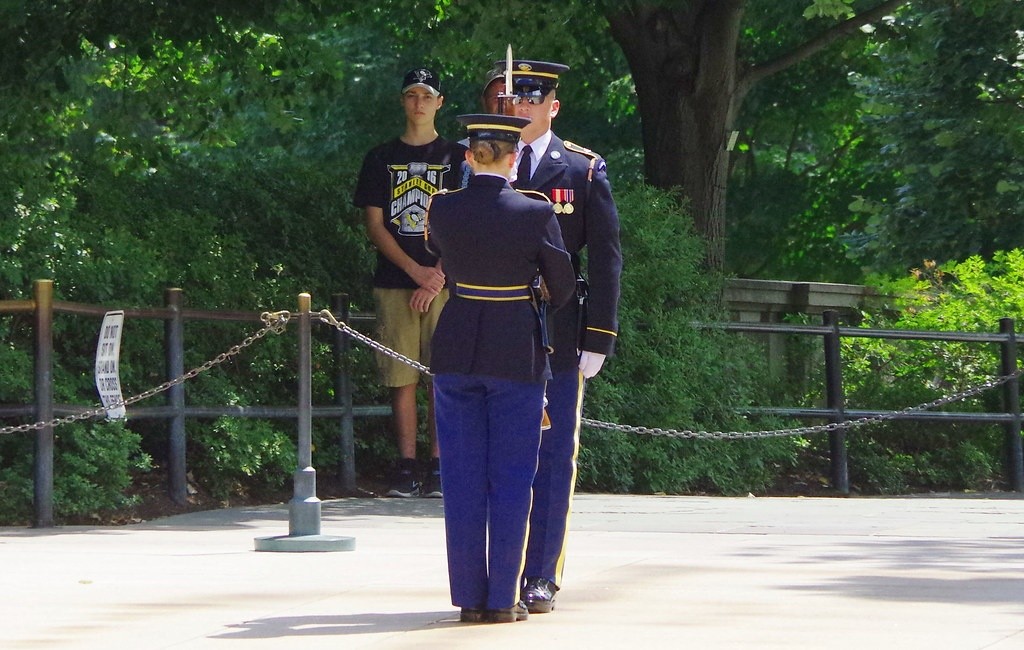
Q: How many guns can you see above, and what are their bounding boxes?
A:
[497,43,553,430]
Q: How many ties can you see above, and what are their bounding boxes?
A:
[516,145,533,182]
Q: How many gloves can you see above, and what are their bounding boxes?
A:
[578,349,606,379]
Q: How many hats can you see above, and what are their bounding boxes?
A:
[481,67,505,96]
[494,59,570,98]
[400,66,441,96]
[455,112,532,143]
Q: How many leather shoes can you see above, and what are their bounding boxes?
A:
[519,575,559,613]
[459,600,530,625]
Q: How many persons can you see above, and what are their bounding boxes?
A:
[507,53,621,614]
[424,114,577,622]
[352,69,471,498]
[482,64,509,116]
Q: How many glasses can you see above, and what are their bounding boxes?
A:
[511,95,546,106]
